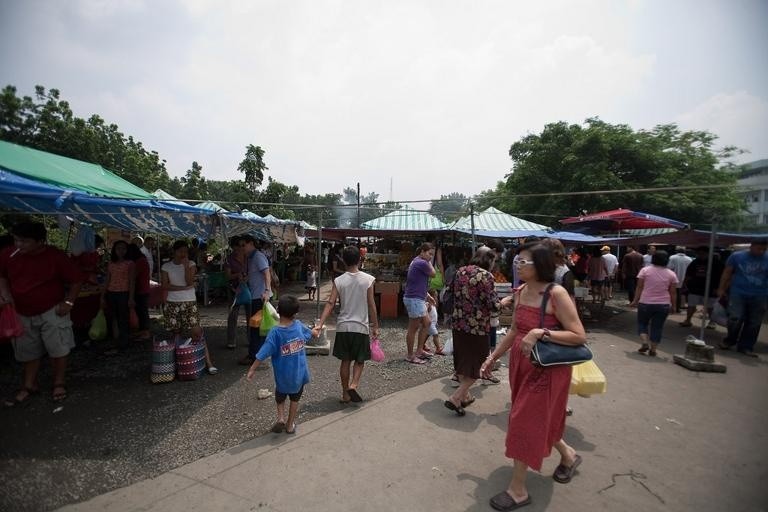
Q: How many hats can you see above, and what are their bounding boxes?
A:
[144,233,155,240]
[131,236,144,246]
[599,245,610,252]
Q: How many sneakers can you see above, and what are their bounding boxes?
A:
[679,320,691,327]
[707,322,716,329]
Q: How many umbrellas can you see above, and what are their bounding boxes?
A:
[559,207,688,259]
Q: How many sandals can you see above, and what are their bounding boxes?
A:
[714,342,760,358]
[638,344,657,356]
[50,383,68,403]
[3,382,41,408]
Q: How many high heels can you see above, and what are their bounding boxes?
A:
[450,374,460,388]
[481,368,501,386]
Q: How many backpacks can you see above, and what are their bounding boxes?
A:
[428,265,444,291]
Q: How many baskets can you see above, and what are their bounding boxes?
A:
[175,341,208,382]
[149,341,177,384]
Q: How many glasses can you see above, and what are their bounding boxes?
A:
[512,260,534,266]
[239,244,245,248]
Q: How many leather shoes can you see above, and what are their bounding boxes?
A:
[238,355,250,366]
[225,343,236,350]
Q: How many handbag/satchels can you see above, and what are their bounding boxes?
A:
[710,297,729,326]
[235,281,252,306]
[442,288,456,314]
[527,281,593,370]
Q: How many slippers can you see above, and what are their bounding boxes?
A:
[552,453,583,484]
[444,394,476,417]
[489,489,532,511]
[405,349,434,364]
[272,419,297,434]
[339,386,363,403]
[436,346,447,356]
[207,366,220,376]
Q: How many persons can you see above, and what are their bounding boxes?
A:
[441,246,513,416]
[244,295,318,434]
[2,217,764,410]
[478,240,587,511]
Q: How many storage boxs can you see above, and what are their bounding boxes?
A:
[374,282,402,294]
